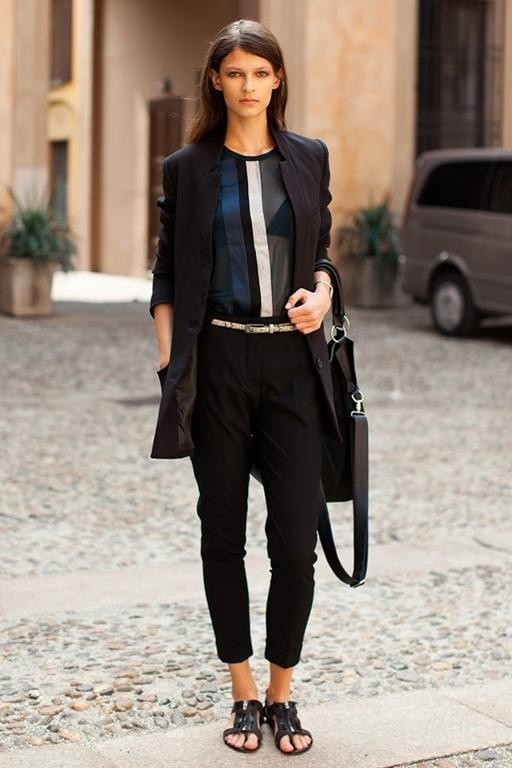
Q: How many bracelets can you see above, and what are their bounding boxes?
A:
[314,279,334,298]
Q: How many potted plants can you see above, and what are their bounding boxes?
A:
[338,189,402,306]
[1,183,79,317]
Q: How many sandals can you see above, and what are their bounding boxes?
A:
[264,698,313,755]
[224,700,264,752]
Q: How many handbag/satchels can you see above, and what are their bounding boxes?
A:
[314,258,368,500]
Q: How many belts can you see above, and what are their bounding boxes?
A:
[211,319,296,334]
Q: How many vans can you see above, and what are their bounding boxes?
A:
[402,147,511,338]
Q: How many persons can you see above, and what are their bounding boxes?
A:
[146,19,335,754]
